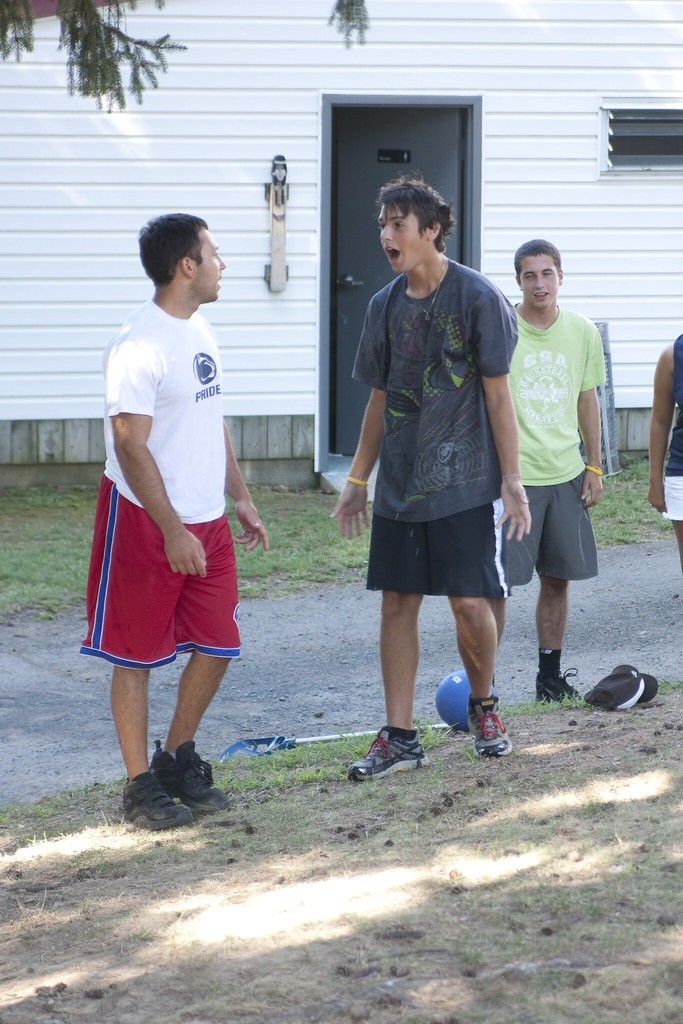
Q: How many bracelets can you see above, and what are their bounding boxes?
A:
[347,476,369,487]
[585,466,603,475]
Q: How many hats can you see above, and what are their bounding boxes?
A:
[220,735,298,763]
[584,665,658,711]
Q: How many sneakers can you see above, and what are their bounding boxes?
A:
[149,740,230,812]
[468,688,512,761]
[347,729,429,782]
[122,772,193,831]
[535,667,583,707]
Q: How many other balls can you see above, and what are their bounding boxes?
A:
[434,668,499,732]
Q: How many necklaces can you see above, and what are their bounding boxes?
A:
[417,255,445,320]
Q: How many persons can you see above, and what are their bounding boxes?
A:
[487,238,608,705]
[77,214,272,827]
[648,334,683,569]
[328,180,532,780]
